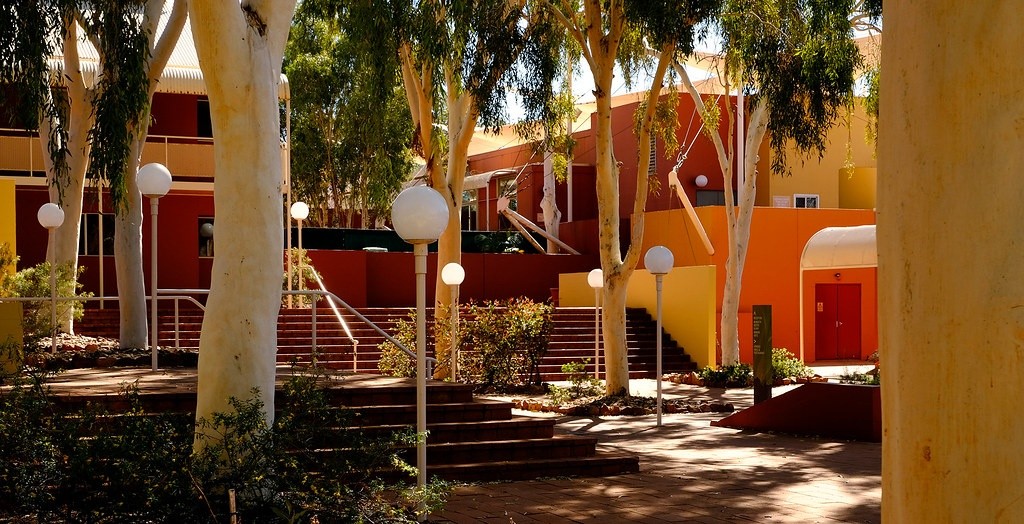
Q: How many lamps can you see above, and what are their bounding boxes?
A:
[695,175,708,186]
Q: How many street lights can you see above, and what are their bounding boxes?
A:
[391,185,451,521]
[289,201,309,308]
[37,203,65,354]
[442,262,466,383]
[135,163,172,370]
[587,268,604,379]
[642,245,674,427]
